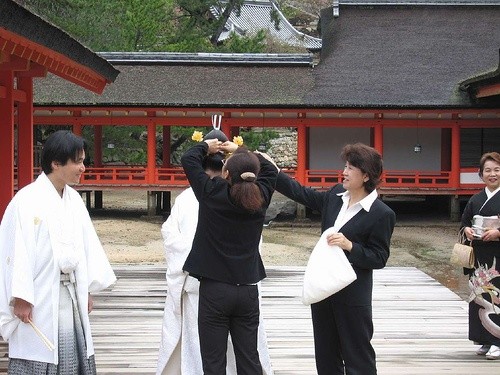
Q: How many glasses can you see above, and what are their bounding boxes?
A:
[222,158,232,176]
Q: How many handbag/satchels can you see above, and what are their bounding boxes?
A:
[450,233,474,269]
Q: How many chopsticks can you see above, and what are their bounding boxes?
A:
[28,319,55,352]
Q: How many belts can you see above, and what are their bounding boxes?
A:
[59,274,71,282]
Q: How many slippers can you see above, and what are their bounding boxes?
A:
[477,344,491,354]
[486,345,500,360]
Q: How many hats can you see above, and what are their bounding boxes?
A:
[202,130,228,144]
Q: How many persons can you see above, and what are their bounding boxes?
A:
[459,152,500,360]
[181,138,278,375]
[155,128,275,375]
[254,143,396,375]
[0,130,118,375]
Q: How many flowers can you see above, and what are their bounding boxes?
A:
[233,135,243,147]
[192,130,204,142]
